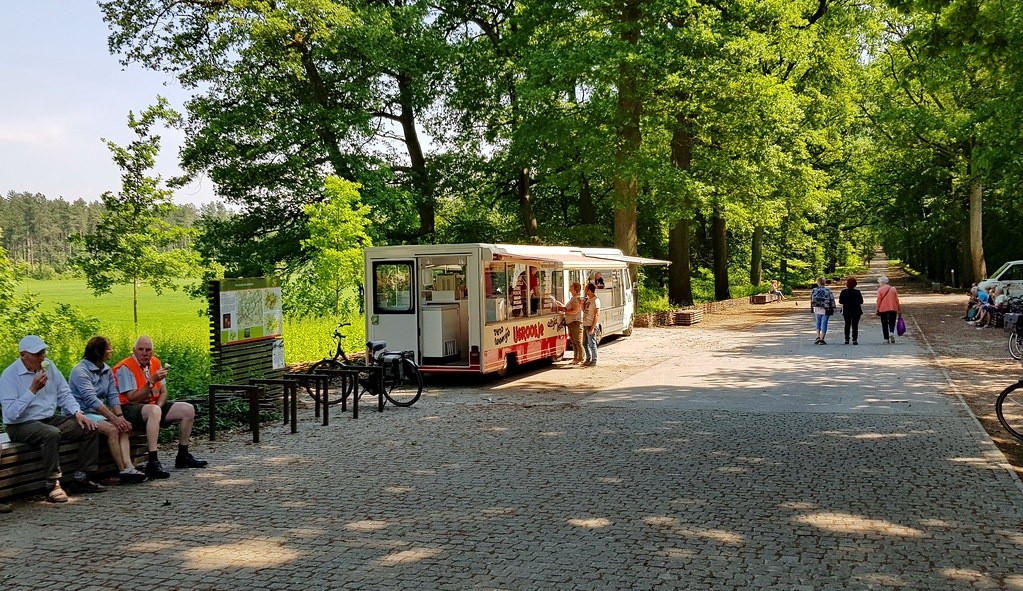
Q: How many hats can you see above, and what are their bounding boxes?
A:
[19,335,49,355]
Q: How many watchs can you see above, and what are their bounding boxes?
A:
[147,381,154,388]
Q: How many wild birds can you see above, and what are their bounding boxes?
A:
[796,302,798,306]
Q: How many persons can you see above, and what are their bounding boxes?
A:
[111,336,208,479]
[577,283,600,366]
[975,288,1008,328]
[838,277,864,345]
[975,287,997,328]
[810,276,838,345]
[225,315,230,327]
[966,286,989,326]
[512,265,539,318]
[588,270,604,289]
[771,280,788,303]
[0,334,108,502]
[68,337,149,483]
[875,274,901,344]
[962,287,978,320]
[550,282,585,365]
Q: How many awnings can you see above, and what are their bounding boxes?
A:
[497,253,672,269]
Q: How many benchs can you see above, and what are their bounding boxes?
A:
[672,309,704,325]
[754,292,781,304]
[985,312,1004,328]
[0,405,164,502]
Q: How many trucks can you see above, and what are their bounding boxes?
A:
[361,243,672,388]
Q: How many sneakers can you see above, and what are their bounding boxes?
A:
[119,465,150,482]
[47,480,68,502]
[146,461,170,478]
[71,477,108,492]
[175,453,208,468]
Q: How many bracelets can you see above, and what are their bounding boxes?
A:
[116,414,124,416]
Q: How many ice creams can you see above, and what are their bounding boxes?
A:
[164,362,171,370]
[41,361,49,373]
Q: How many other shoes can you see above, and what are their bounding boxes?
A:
[0,503,14,513]
[580,361,596,368]
[853,341,858,345]
[569,358,583,365]
[819,340,826,345]
[845,341,849,344]
[889,332,895,343]
[882,340,888,344]
[962,314,990,329]
[814,337,820,343]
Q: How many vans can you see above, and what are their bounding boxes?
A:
[976,260,1023,302]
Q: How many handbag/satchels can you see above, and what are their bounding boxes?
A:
[813,306,826,315]
[897,314,906,337]
[381,349,415,382]
[840,307,844,316]
[1003,312,1023,334]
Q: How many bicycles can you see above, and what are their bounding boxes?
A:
[305,322,424,407]
[995,380,1023,441]
[1006,294,1023,360]
